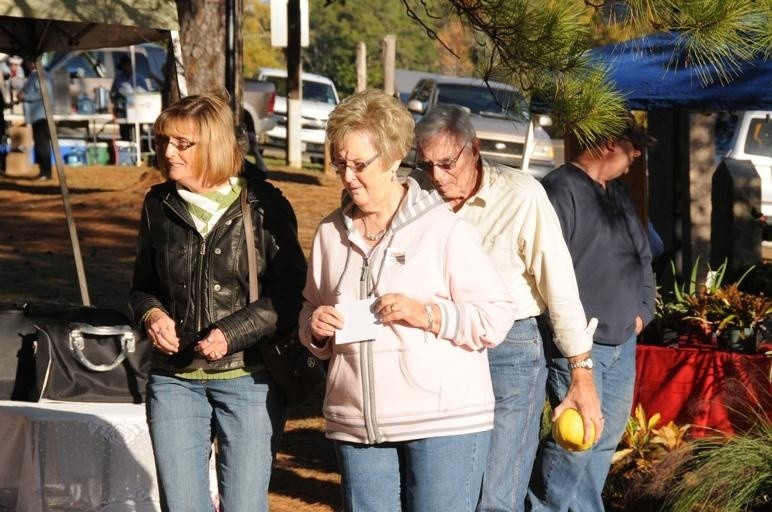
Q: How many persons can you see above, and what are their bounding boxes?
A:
[403,100,606,512]
[5,54,25,114]
[296,87,523,512]
[110,57,148,144]
[17,55,57,182]
[539,110,659,512]
[126,91,308,512]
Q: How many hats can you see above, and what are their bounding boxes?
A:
[619,111,656,147]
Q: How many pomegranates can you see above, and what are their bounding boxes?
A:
[552,405,597,453]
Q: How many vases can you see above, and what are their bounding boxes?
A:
[716,328,760,352]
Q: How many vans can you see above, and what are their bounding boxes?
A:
[711,108,772,242]
[247,67,341,166]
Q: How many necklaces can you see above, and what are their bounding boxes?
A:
[358,213,386,241]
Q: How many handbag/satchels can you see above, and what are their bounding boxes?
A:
[263,333,325,408]
[33,316,149,402]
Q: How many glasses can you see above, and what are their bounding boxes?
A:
[329,153,378,171]
[416,145,464,170]
[155,137,195,150]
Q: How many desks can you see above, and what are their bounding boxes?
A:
[630,345,772,442]
[0,398,225,512]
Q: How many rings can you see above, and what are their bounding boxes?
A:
[214,350,219,357]
[390,303,396,312]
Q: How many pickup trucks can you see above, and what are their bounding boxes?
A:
[389,67,556,183]
[45,43,278,158]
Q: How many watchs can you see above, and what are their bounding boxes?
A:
[568,356,594,370]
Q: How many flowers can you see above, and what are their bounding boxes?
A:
[678,282,772,343]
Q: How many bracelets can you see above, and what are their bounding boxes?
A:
[424,302,434,332]
